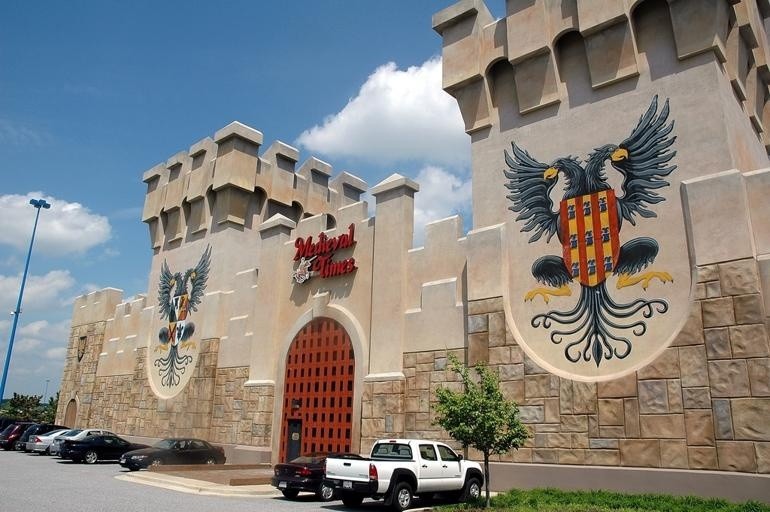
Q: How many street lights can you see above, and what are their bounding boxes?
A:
[0,197,52,407]
[43,379,50,403]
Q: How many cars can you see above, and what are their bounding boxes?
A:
[270,450,367,501]
[117,436,228,471]
[0,421,153,464]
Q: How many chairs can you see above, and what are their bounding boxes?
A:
[378,448,388,454]
[390,451,398,455]
[400,449,409,455]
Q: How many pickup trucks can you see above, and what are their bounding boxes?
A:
[321,437,485,512]
